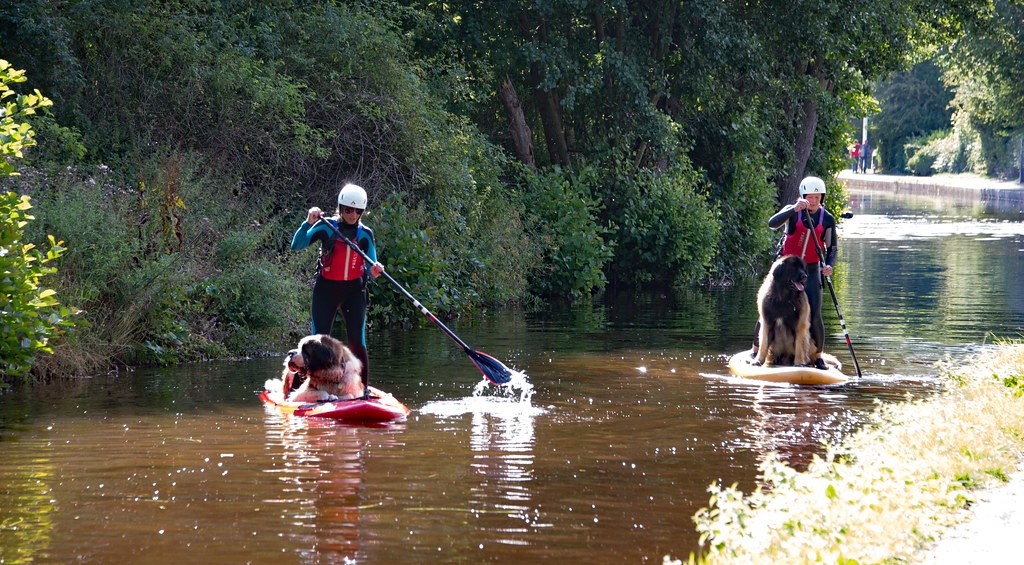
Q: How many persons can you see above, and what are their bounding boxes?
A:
[291,185,385,397]
[849,139,861,173]
[859,140,869,174]
[872,149,878,170]
[746,175,837,370]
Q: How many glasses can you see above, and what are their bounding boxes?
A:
[340,205,364,215]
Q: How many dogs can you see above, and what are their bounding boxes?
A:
[748,253,842,369]
[264,334,367,403]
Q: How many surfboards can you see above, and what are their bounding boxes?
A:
[727,345,848,386]
[262,379,408,424]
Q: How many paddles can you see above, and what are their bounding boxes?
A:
[805,204,863,378]
[319,211,513,388]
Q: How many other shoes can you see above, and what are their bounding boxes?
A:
[853,170,857,173]
[750,352,757,358]
[814,358,829,370]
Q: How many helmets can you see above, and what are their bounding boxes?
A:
[337,184,367,218]
[799,176,827,203]
